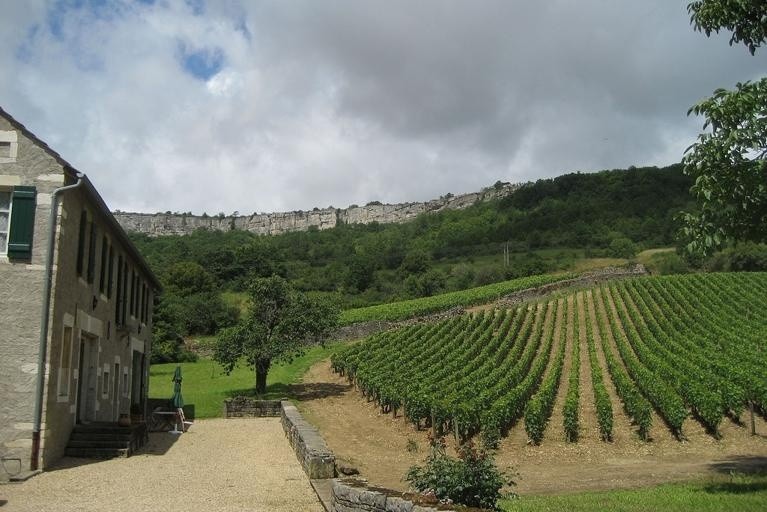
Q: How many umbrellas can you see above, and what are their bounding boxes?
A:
[172,366,184,431]
